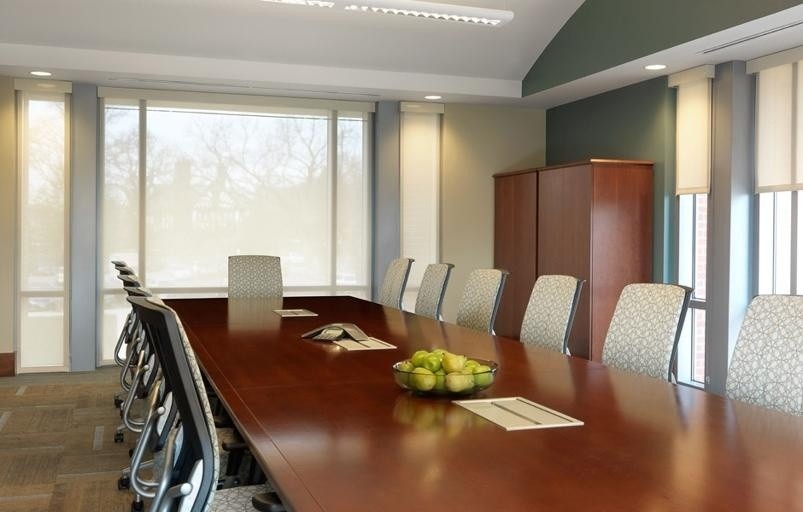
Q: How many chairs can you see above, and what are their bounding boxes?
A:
[109,256,282,510]
[451,266,514,336]
[374,256,416,311]
[596,280,696,386]
[226,255,287,300]
[719,292,802,418]
[516,273,588,358]
[414,260,456,322]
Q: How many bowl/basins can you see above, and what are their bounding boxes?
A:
[391,357,499,398]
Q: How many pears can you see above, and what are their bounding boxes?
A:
[397,348,495,393]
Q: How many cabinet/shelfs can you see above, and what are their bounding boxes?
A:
[493,157,653,362]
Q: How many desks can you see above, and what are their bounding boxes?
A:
[155,291,801,510]
